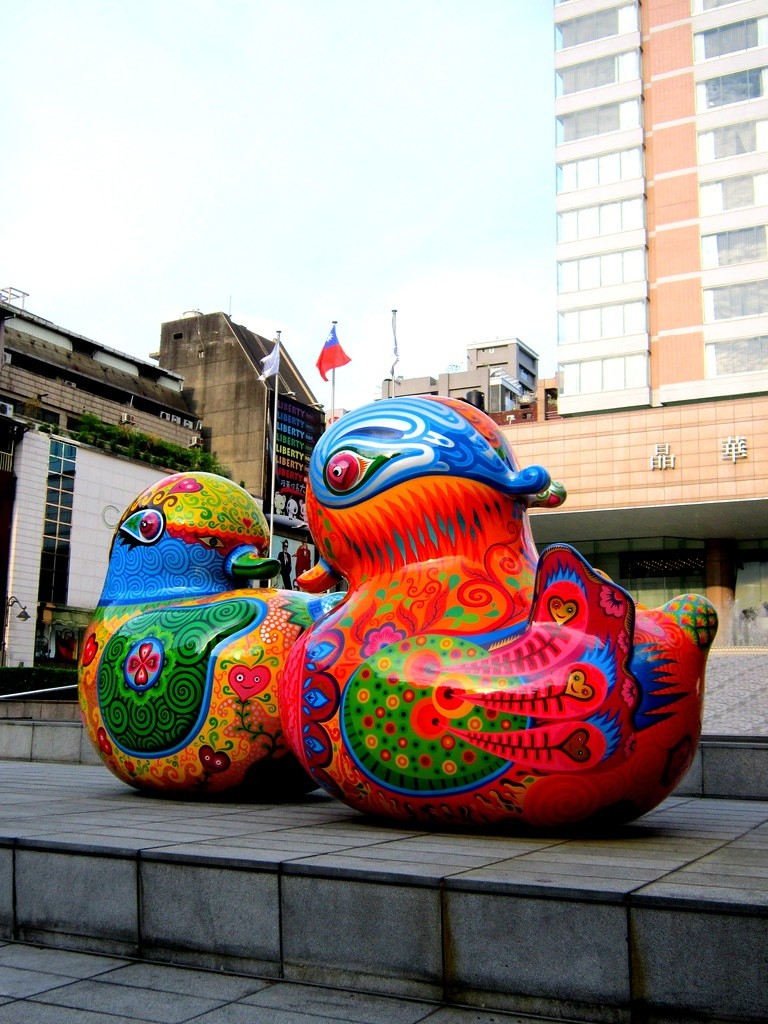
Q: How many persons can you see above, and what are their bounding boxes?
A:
[277,539,292,589]
[294,542,311,578]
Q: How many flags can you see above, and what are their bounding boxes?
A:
[315,325,352,381]
[389,314,400,375]
[257,336,280,381]
